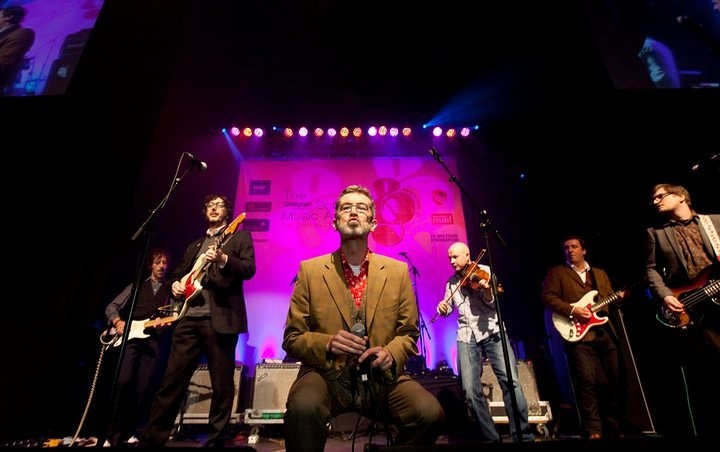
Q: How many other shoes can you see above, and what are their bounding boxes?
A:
[588,432,601,442]
[111,430,124,447]
[124,437,153,448]
[619,430,648,441]
[516,439,537,448]
[488,439,503,451]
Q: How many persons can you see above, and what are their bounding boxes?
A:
[647,182,720,379]
[100,247,171,447]
[281,186,443,452]
[541,233,646,439]
[139,195,257,452]
[437,242,534,444]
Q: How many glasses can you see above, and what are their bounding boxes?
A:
[205,202,226,208]
[650,192,671,207]
[337,204,368,213]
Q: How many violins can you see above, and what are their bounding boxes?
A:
[461,263,504,294]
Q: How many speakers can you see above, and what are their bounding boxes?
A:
[249,364,303,425]
[174,360,244,423]
[480,361,553,424]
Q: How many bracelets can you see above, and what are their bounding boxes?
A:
[115,319,121,326]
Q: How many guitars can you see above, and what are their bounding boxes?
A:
[661,262,720,329]
[172,211,247,319]
[552,287,626,342]
[111,315,174,348]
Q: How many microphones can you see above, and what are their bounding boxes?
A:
[350,322,371,392]
[184,149,208,172]
